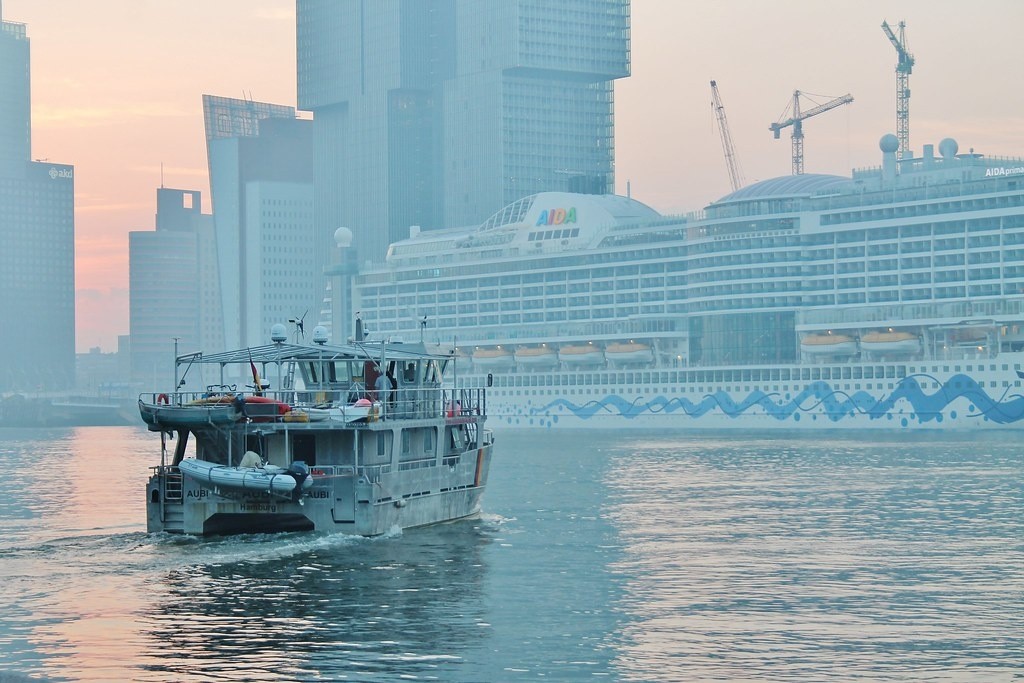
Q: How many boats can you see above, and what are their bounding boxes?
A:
[800,334,856,356]
[603,342,653,363]
[860,331,919,352]
[136,308,497,541]
[473,348,512,364]
[950,318,998,348]
[513,346,555,364]
[447,351,471,364]
[558,345,601,363]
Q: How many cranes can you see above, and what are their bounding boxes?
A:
[768,89,851,176]
[880,19,914,162]
[707,79,740,192]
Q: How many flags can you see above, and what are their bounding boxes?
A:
[250,358,262,391]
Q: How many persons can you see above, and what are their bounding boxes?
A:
[375,369,393,410]
[404,363,415,381]
